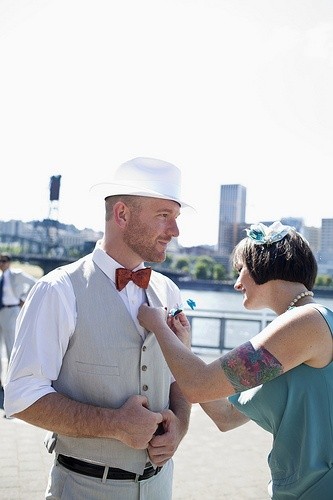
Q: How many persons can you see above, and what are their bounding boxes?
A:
[0,253,36,364]
[3,156,192,500]
[138,222,333,500]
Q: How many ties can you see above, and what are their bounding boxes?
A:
[0,272,4,310]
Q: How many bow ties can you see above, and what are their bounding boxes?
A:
[115,266,152,292]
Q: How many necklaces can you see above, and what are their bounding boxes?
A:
[287,291,314,310]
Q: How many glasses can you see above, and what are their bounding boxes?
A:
[0,259,7,263]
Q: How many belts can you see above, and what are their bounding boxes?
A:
[57,453,163,481]
[3,304,20,308]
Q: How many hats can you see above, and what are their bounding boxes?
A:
[89,157,196,209]
